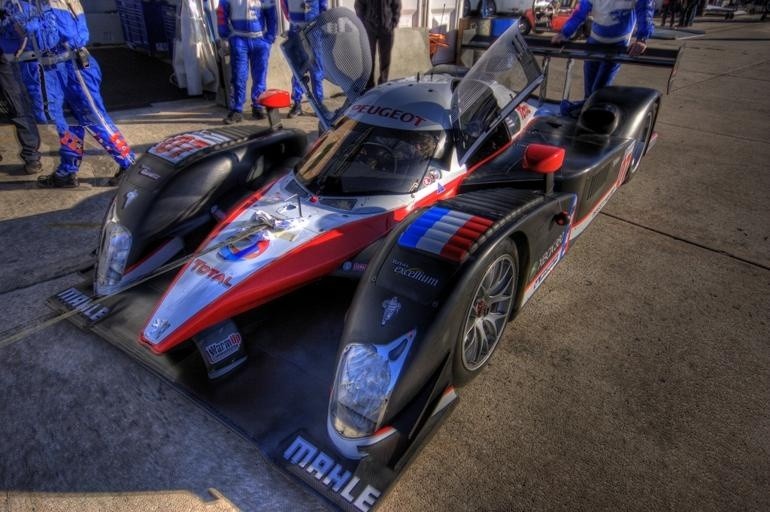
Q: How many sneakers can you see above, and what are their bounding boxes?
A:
[23,157,43,174]
[250,105,267,122]
[109,168,125,186]
[34,173,80,189]
[287,102,305,117]
[223,110,244,124]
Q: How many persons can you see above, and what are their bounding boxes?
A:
[550,0,657,98]
[280,0,330,119]
[0,0,44,175]
[217,1,275,124]
[14,0,137,189]
[354,0,401,99]
[658,1,770,37]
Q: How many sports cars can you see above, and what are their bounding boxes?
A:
[32,14,688,512]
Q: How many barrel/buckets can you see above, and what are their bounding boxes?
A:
[455,16,491,73]
[486,12,521,73]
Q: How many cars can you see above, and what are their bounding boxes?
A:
[744,0,766,13]
[463,0,581,36]
